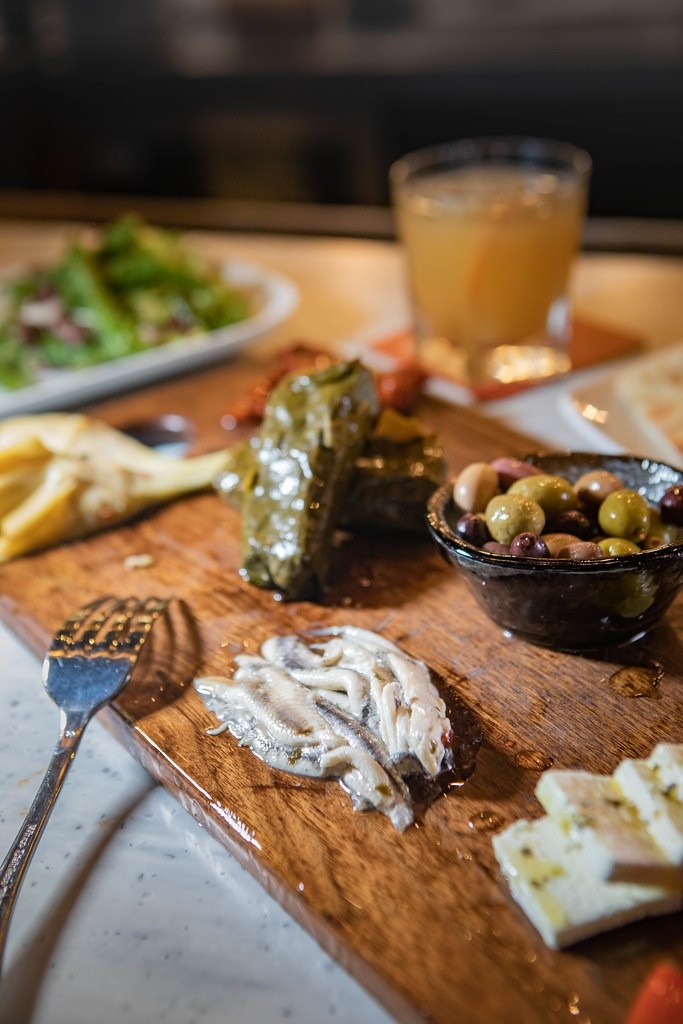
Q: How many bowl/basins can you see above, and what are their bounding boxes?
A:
[426,451,683,648]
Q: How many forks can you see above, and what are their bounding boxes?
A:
[1,594,163,963]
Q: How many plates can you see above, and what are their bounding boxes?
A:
[0,260,303,416]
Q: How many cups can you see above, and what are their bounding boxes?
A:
[390,136,582,360]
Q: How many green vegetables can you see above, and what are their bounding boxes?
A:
[0,209,260,396]
